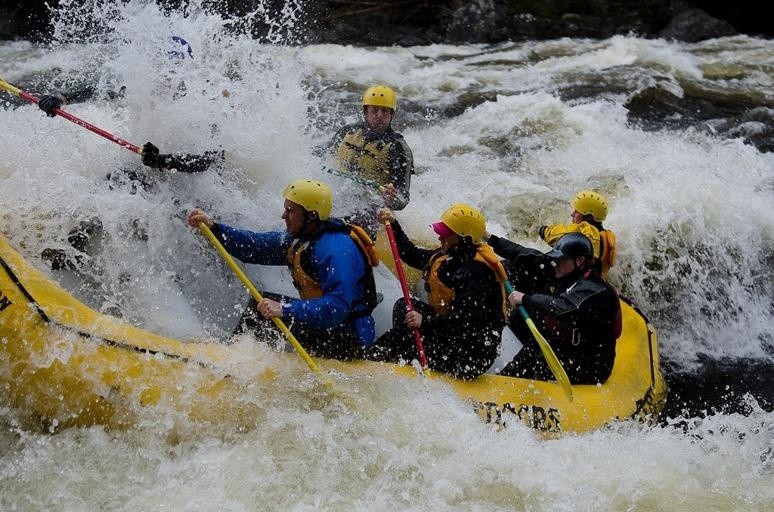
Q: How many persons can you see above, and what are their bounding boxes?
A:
[188,180,377,364]
[314,85,414,238]
[538,190,617,282]
[375,203,508,380]
[38,34,224,171]
[478,231,622,384]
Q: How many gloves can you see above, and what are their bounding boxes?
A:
[39,95,63,117]
[141,142,160,167]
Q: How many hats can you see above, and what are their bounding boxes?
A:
[429,222,457,236]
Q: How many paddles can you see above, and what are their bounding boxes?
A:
[495,261,576,403]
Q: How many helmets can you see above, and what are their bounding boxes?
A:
[363,87,396,112]
[569,191,609,223]
[440,204,486,245]
[159,36,193,62]
[544,232,594,259]
[283,180,333,221]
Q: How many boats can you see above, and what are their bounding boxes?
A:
[0,117,668,454]
